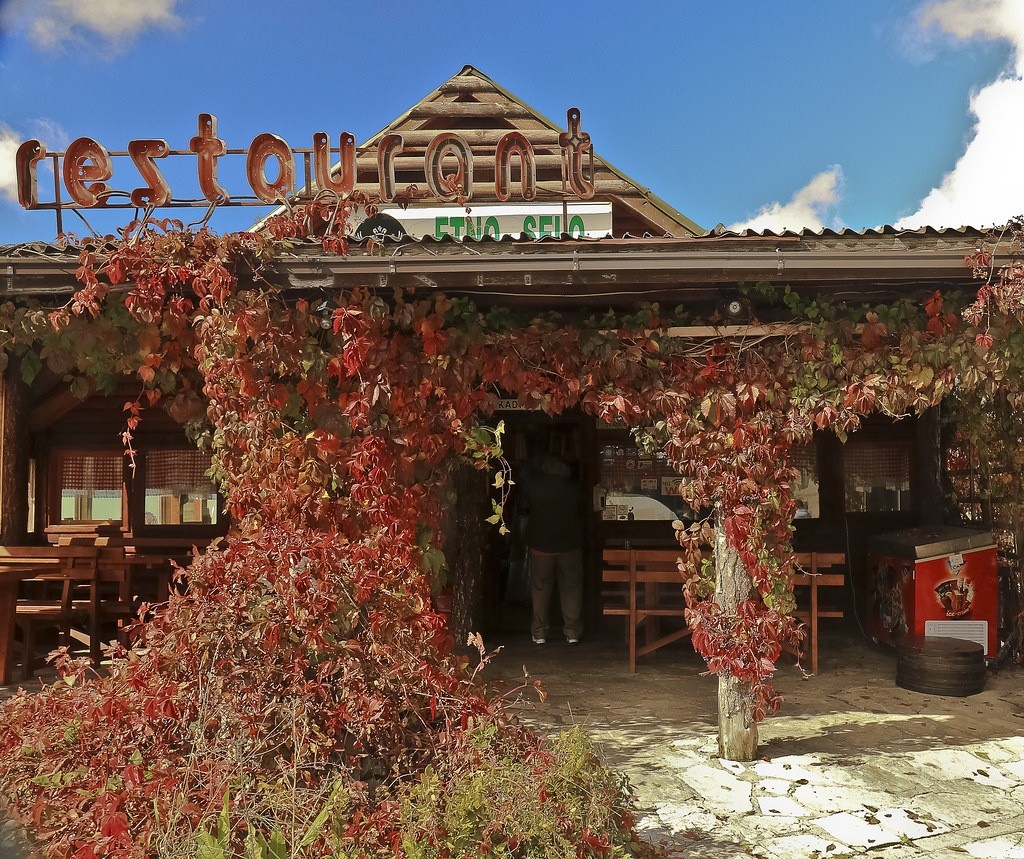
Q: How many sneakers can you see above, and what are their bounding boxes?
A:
[569,639,578,644]
[532,636,545,643]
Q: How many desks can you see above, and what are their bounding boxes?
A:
[599,531,849,658]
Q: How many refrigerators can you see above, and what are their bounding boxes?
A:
[863,523,999,660]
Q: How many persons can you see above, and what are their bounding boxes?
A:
[519,450,588,645]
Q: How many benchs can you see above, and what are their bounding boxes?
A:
[601,548,847,676]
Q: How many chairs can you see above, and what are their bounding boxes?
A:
[0,535,229,687]
